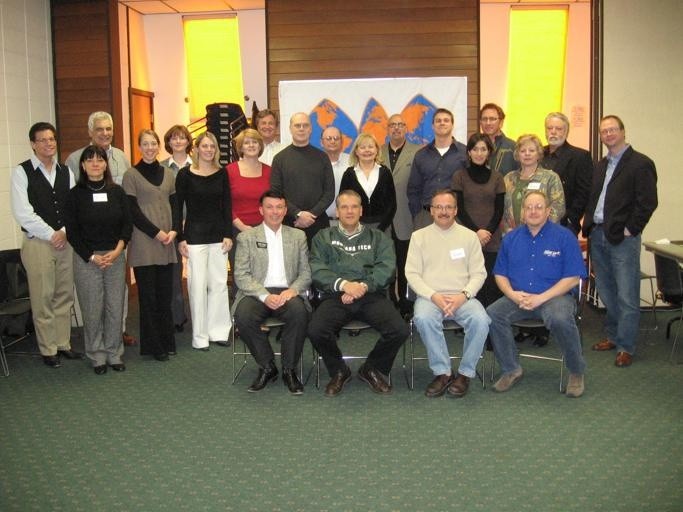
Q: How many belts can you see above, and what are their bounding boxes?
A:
[328,216,340,220]
[423,205,430,210]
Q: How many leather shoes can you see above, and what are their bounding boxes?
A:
[325,366,353,397]
[358,363,392,394]
[282,367,305,395]
[592,341,610,351]
[616,351,632,366]
[42,333,177,375]
[425,369,454,398]
[447,374,470,396]
[515,333,527,343]
[247,366,279,393]
[533,335,549,347]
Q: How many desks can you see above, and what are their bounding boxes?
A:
[642,229,682,298]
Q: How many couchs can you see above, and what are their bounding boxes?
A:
[230,312,315,387]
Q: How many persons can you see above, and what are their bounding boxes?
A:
[230,189,311,394]
[580,116,658,368]
[10,122,84,369]
[63,101,593,361]
[405,191,492,397]
[308,191,409,397]
[65,146,133,376]
[484,190,589,399]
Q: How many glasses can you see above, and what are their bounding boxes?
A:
[524,204,545,211]
[322,135,340,140]
[430,205,456,214]
[388,123,405,129]
[35,137,56,144]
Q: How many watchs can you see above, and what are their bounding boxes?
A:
[465,292,473,303]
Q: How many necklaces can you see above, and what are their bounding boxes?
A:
[85,181,106,191]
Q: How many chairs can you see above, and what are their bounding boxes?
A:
[654,235,683,364]
[205,102,249,163]
[403,281,488,389]
[316,264,399,389]
[0,248,81,379]
[630,261,662,331]
[488,277,582,392]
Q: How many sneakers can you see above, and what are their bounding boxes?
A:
[566,373,584,397]
[491,368,523,393]
